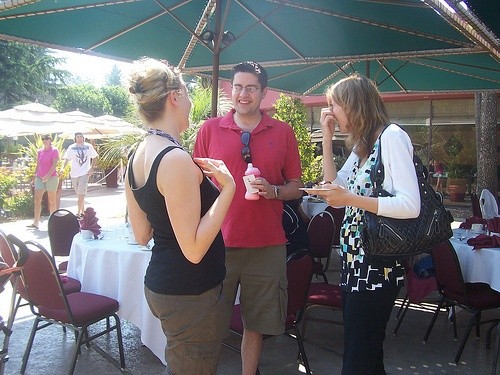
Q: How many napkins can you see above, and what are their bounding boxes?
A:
[467,234,500,249]
[485,217,500,233]
[78,206,102,238]
[459,216,486,229]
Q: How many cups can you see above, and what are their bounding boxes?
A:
[81,223,154,249]
[472,224,483,232]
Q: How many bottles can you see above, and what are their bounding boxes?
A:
[244,162,261,200]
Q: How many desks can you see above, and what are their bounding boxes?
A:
[66,224,167,365]
[448,228,500,292]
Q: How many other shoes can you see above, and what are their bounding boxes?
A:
[75,213,84,220]
[27,224,39,229]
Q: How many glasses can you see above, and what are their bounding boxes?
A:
[42,138,51,140]
[231,85,261,93]
[241,131,251,162]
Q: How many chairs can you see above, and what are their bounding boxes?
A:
[225,206,500,375]
[0,209,130,375]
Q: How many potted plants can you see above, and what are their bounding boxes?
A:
[20,132,69,210]
[100,155,122,188]
[446,172,470,203]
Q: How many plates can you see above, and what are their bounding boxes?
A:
[141,247,152,251]
[127,242,140,245]
[299,187,330,192]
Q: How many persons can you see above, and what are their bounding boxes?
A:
[26,133,60,228]
[125,59,236,375]
[193,62,303,375]
[58,132,98,218]
[307,76,421,375]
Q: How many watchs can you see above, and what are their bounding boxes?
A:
[272,185,280,199]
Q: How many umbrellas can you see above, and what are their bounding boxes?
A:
[0,0,500,118]
[0,102,147,141]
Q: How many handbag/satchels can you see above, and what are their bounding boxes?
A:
[365,122,453,256]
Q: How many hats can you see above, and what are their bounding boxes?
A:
[42,133,52,138]
